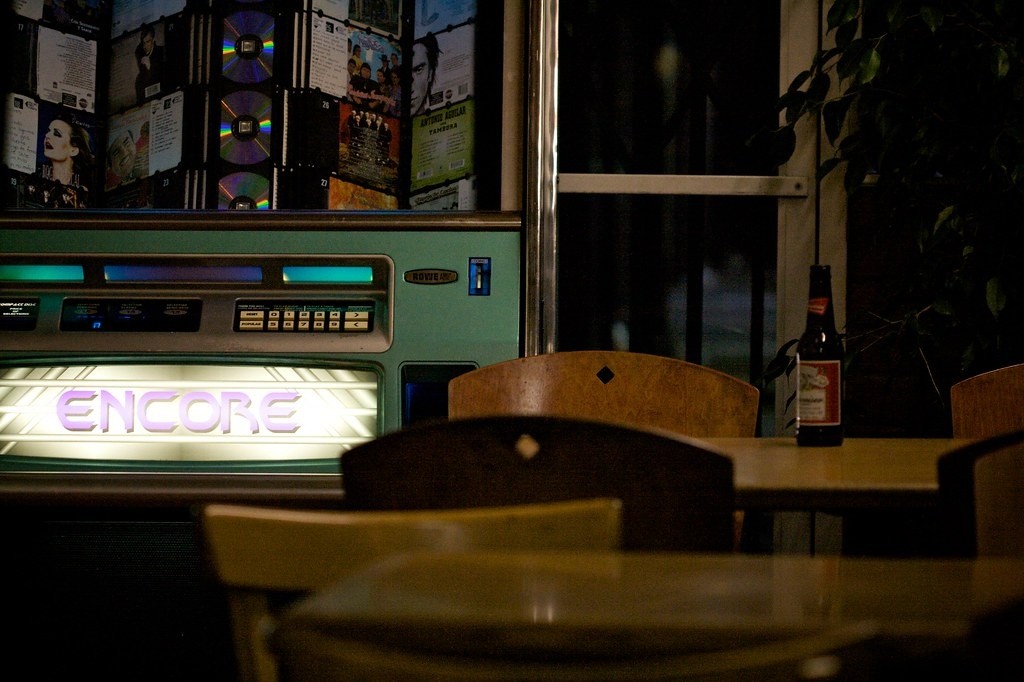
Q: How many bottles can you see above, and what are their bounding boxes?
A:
[795,263,845,448]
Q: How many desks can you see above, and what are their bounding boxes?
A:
[702,435,982,558]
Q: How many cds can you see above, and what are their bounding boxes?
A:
[217,172,270,210]
[223,10,275,82]
[220,91,273,164]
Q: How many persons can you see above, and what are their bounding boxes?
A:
[25,184,73,209]
[140,26,166,102]
[38,116,95,188]
[347,110,392,148]
[107,129,149,189]
[348,38,401,117]
[410,36,444,116]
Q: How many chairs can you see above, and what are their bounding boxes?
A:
[199,348,1024,682]
